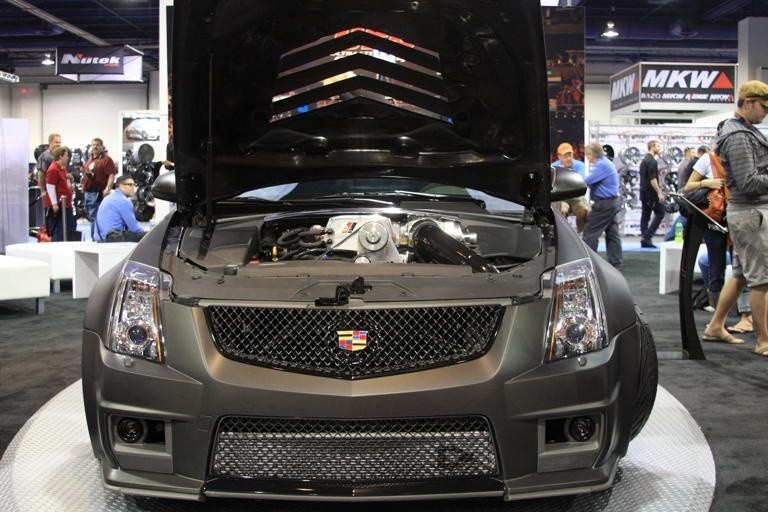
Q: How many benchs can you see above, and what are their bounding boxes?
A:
[5,239,95,293]
[0,254,51,314]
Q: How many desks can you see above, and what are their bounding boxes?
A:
[71,241,139,299]
[659,239,710,295]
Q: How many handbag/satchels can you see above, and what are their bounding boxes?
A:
[700,189,725,223]
[679,188,710,218]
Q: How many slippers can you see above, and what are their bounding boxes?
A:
[703,325,768,357]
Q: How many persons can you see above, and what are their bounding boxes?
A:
[637,139,666,251]
[682,151,756,334]
[549,142,586,180]
[582,142,625,272]
[662,146,696,242]
[686,145,710,181]
[701,79,768,359]
[35,133,149,243]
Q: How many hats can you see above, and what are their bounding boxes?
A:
[558,143,573,155]
[739,80,768,107]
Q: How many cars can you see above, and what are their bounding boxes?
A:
[123,119,161,140]
[80,0,660,504]
